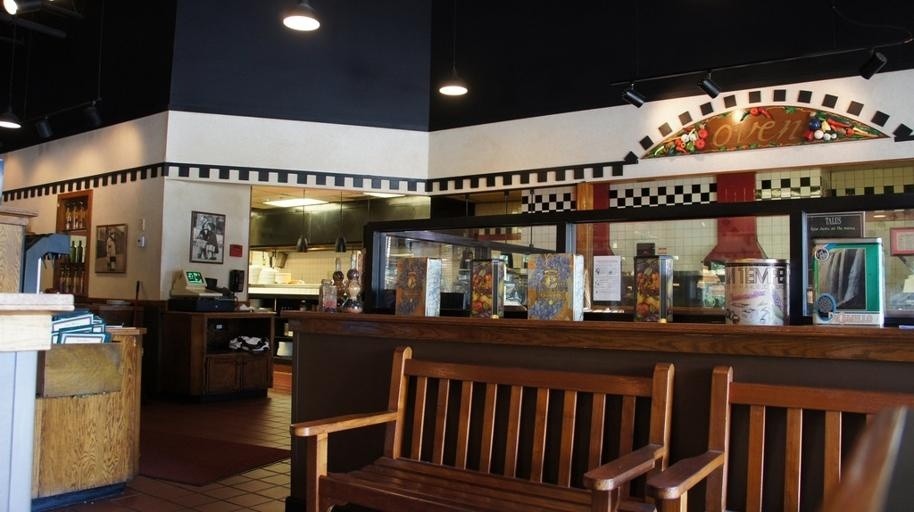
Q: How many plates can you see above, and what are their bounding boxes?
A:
[275,273,291,282]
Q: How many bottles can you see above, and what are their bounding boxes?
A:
[320,270,364,312]
[66,200,86,231]
[57,241,83,296]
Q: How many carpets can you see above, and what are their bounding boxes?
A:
[139,434,290,486]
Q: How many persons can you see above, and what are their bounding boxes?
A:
[105,229,117,271]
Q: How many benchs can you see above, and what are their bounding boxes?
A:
[647,368,910,511]
[290,346,676,512]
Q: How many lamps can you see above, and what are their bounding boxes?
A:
[610,42,907,107]
[283,4,319,32]
[295,188,308,253]
[438,13,468,97]
[334,190,347,252]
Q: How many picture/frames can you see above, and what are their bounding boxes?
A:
[889,227,914,256]
[188,210,226,265]
[95,223,128,274]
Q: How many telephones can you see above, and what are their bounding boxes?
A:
[230,270,244,292]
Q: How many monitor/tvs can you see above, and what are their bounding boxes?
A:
[173,269,207,290]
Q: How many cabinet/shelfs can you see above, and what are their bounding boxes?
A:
[162,310,275,405]
[247,294,318,365]
[52,189,93,298]
